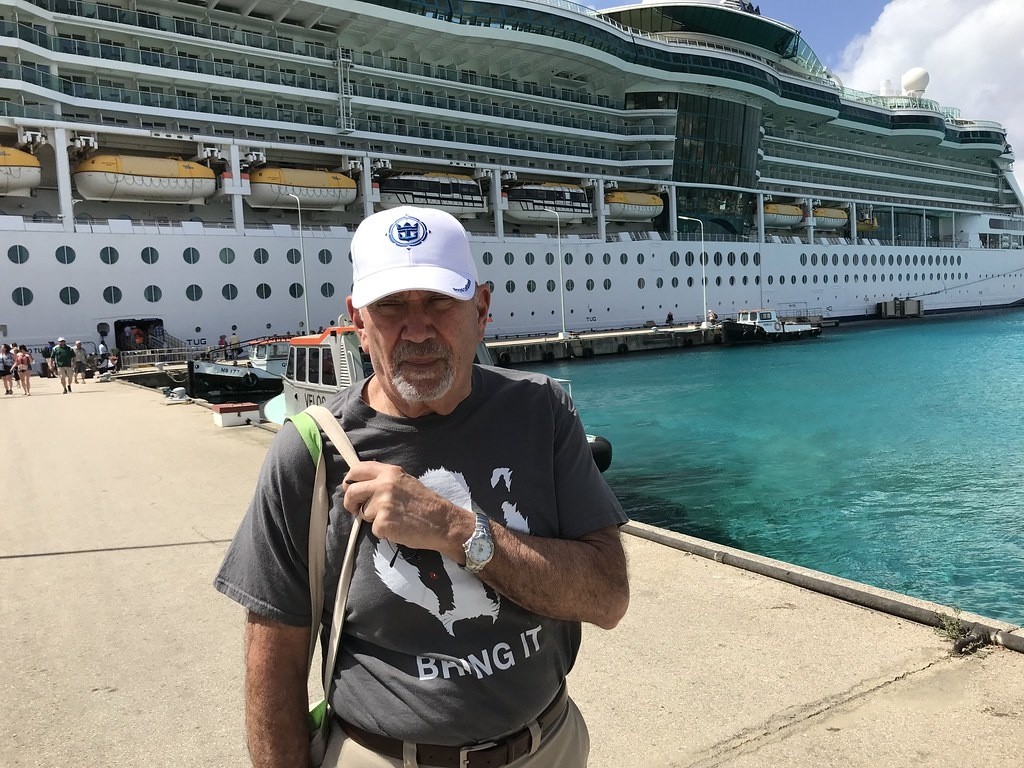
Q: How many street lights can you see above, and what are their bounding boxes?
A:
[678,216,707,322]
[544,209,565,332]
[284,192,310,336]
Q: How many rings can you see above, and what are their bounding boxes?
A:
[359,504,373,520]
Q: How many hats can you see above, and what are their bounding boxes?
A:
[349,205,477,308]
[58,337,66,342]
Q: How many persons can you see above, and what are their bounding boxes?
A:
[148,324,164,341]
[667,311,674,328]
[46,341,57,378]
[317,326,322,334]
[0,343,33,396]
[213,204,630,768]
[229,332,240,359]
[97,340,116,375]
[707,309,715,325]
[49,337,77,394]
[72,341,85,384]
[218,334,227,348]
[124,325,144,345]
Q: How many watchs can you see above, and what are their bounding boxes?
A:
[457,513,494,573]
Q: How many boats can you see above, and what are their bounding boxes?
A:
[604,192,663,222]
[856,217,879,232]
[0,147,41,197]
[184,336,304,404]
[258,315,612,473]
[753,204,803,229]
[721,309,822,343]
[245,168,357,212]
[379,173,488,219]
[503,181,593,226]
[72,154,216,206]
[813,208,848,231]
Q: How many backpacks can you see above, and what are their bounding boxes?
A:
[42,347,52,358]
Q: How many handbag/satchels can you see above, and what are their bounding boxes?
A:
[308,697,329,768]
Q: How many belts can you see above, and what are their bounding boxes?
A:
[331,678,568,768]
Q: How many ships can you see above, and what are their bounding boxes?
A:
[0,0,1024,376]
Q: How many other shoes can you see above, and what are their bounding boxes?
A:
[68,385,71,392]
[9,389,13,394]
[5,390,9,395]
[63,388,67,394]
